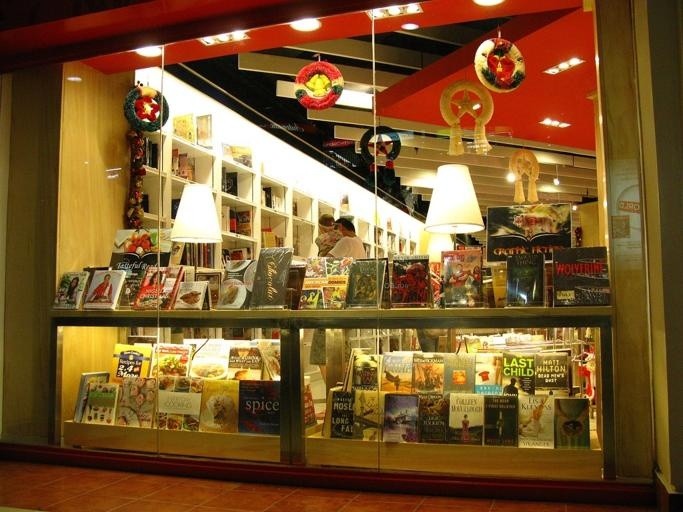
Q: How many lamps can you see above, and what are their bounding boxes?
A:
[424,161,487,254]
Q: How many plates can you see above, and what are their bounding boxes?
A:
[243,260,259,293]
[219,279,247,309]
[190,357,227,379]
[226,260,252,273]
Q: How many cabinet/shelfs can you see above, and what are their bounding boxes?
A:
[143,125,415,263]
[52,303,618,478]
